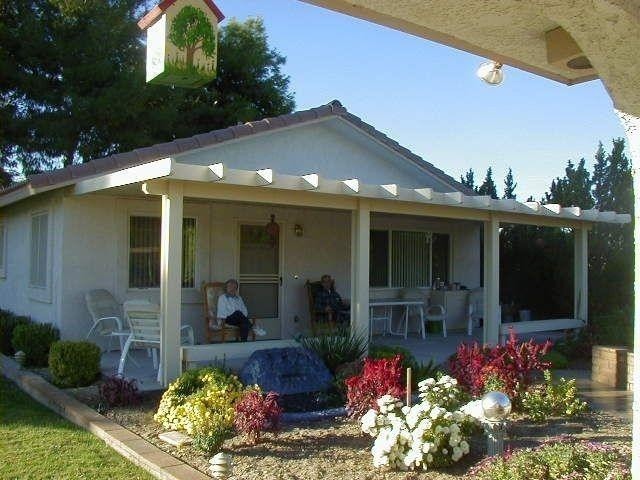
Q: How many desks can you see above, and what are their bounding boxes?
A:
[368,297,427,344]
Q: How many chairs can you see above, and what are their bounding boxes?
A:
[201,280,256,343]
[397,288,449,339]
[303,278,349,337]
[83,289,194,379]
[466,287,501,336]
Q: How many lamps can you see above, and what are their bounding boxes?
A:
[478,61,504,85]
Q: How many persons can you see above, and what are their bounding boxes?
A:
[313,273,352,326]
[216,278,265,343]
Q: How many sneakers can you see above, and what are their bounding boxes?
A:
[252,326,267,336]
